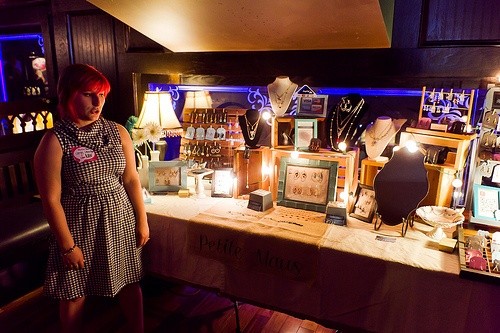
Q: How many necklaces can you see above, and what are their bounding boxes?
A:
[244,82,393,153]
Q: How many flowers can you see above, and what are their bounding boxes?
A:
[134,122,162,151]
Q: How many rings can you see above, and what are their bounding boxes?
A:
[148,238,150,239]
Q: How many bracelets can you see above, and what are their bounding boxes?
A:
[60,244,77,256]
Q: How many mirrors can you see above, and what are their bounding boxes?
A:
[374,144,430,236]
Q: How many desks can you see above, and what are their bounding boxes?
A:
[142,194,500,333]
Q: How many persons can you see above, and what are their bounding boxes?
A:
[32,63,150,333]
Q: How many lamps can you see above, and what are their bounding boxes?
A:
[135,91,182,160]
[180,91,212,120]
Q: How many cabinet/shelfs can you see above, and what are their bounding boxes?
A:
[180,108,246,171]
[398,87,479,209]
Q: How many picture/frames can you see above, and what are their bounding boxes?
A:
[274,118,295,148]
[349,184,375,222]
[149,162,187,191]
[277,156,339,213]
[475,184,500,219]
[211,168,233,197]
[295,120,317,150]
[298,94,328,117]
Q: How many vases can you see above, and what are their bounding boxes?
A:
[139,151,160,187]
